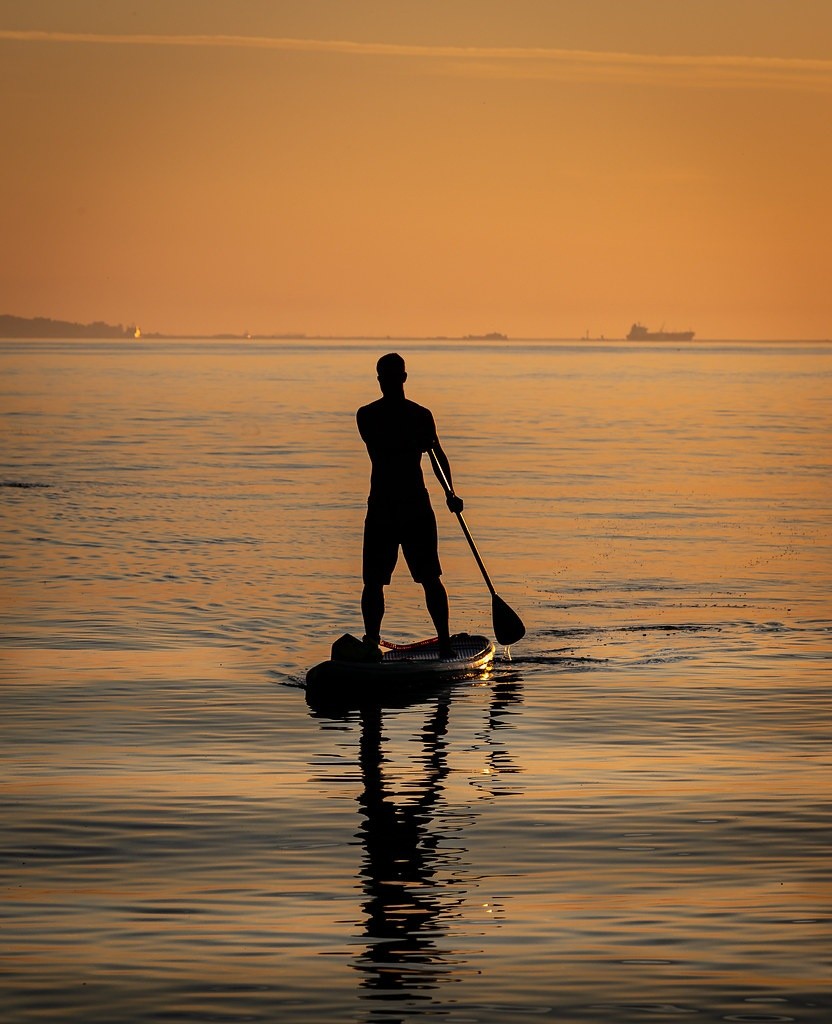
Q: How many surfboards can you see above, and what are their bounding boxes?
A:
[306,632,490,684]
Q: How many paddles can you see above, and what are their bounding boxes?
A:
[427,448,526,646]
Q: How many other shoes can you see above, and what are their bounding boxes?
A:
[437,647,458,659]
[363,635,379,656]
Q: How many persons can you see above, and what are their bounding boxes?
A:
[355,352,464,660]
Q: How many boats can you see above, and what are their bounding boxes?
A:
[625,324,696,341]
